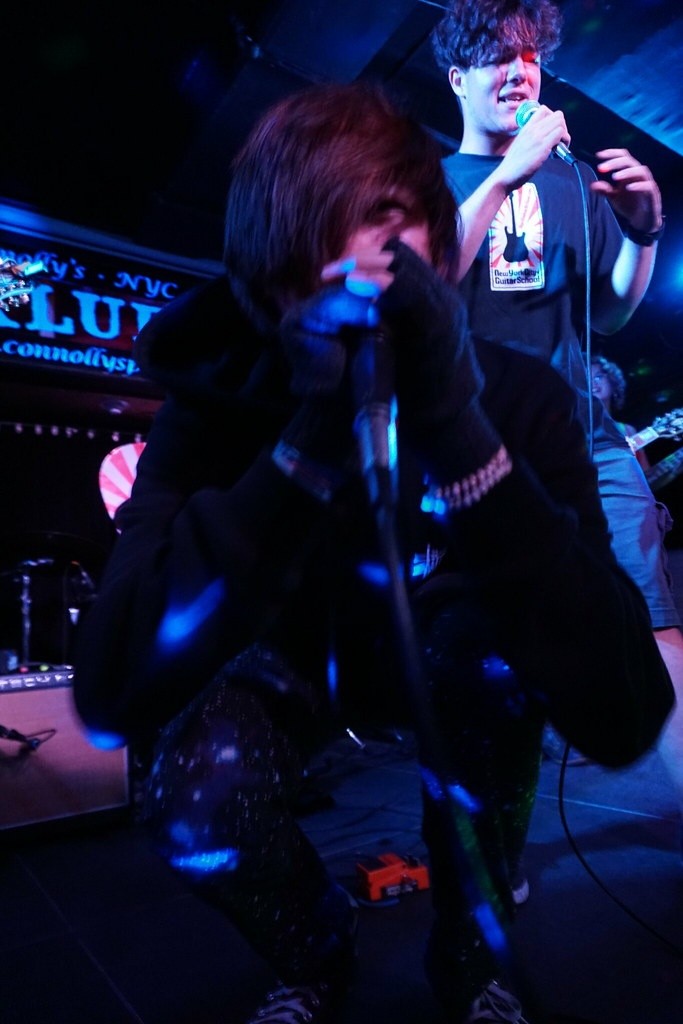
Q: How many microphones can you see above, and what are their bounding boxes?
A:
[19,738,40,756]
[515,98,578,168]
[349,320,398,507]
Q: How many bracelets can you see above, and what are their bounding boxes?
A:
[626,215,667,246]
[436,445,513,508]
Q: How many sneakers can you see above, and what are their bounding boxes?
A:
[253,945,361,1024]
[444,967,531,1024]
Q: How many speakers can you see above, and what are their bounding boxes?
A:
[0,670,137,839]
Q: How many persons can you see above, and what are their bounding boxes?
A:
[74,82,675,1024]
[428,0,683,785]
